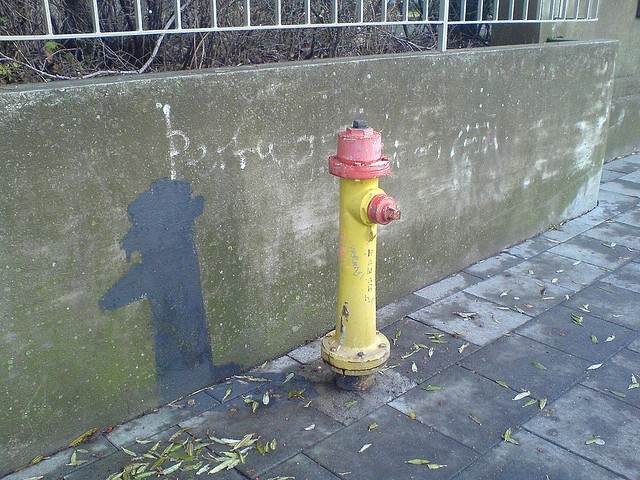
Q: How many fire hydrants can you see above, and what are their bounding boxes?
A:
[96,176,242,412]
[320,118,401,390]
